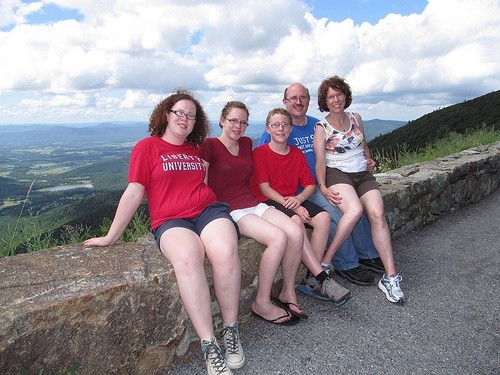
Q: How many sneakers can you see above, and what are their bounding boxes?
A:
[200,336,233,375]
[220,321,246,369]
[378,273,405,304]
[320,277,353,306]
[297,275,332,301]
[320,263,330,276]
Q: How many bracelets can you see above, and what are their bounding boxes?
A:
[319,183,326,189]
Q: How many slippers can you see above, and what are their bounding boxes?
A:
[273,297,309,320]
[253,303,298,326]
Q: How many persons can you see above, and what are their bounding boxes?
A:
[248,108,351,303]
[198,101,308,325]
[82,92,245,375]
[313,77,405,304]
[259,82,385,285]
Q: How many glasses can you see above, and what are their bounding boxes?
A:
[285,96,310,103]
[168,109,197,120]
[267,122,292,128]
[224,117,249,127]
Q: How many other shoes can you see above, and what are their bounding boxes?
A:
[357,257,385,274]
[334,265,375,286]
